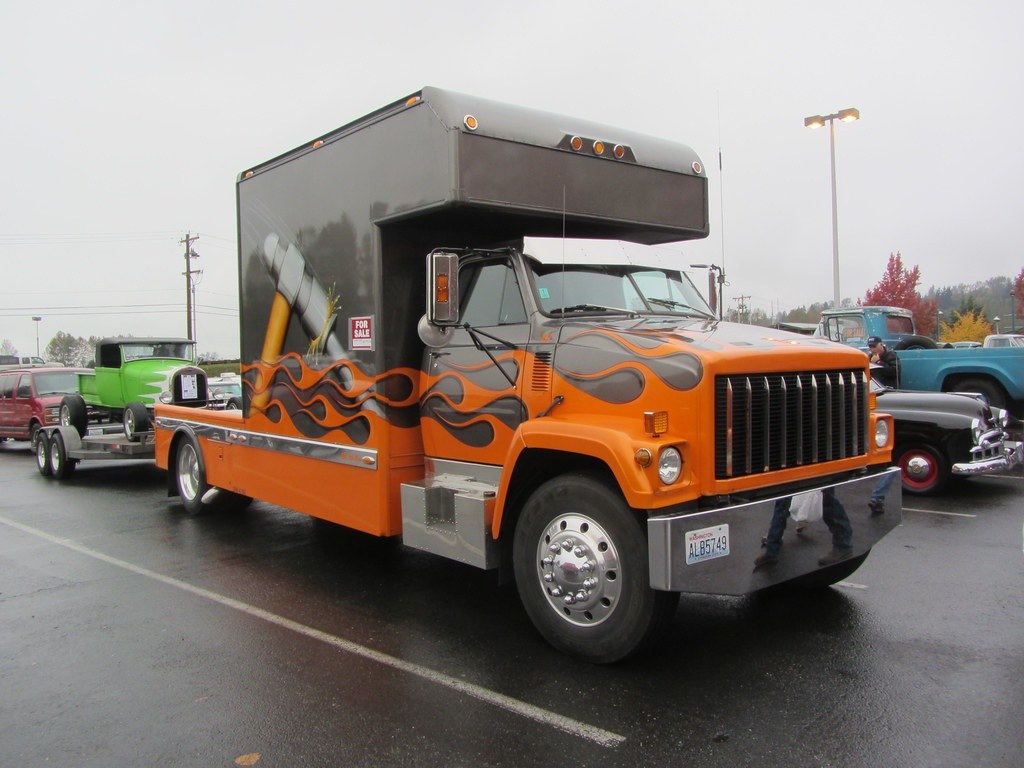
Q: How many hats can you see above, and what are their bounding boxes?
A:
[866,336,881,347]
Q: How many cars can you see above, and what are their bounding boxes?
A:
[206,374,242,401]
[59,335,243,442]
[982,334,1023,348]
[870,367,1023,495]
[0,367,81,452]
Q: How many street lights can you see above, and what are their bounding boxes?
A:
[936,309,943,342]
[993,314,1000,335]
[691,265,723,321]
[803,105,858,309]
[1008,290,1016,334]
[32,316,42,356]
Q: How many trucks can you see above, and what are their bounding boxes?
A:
[813,306,1023,419]
[0,356,65,368]
[152,88,902,663]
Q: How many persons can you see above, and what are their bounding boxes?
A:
[868,472,897,512]
[753,486,853,566]
[866,337,900,389]
